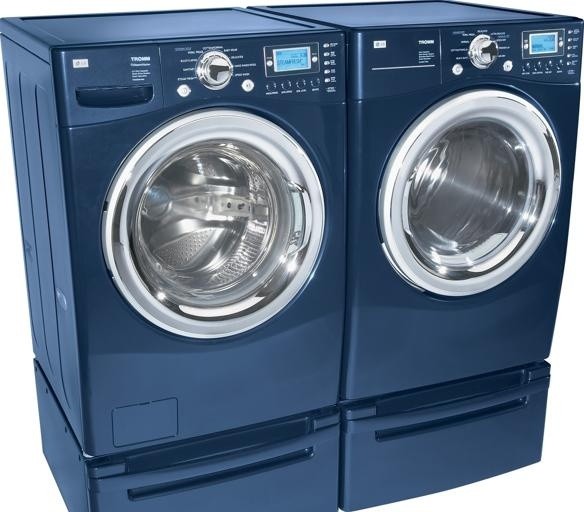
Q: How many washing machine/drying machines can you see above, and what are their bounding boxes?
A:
[0,8,348,511]
[248,0,584,512]
